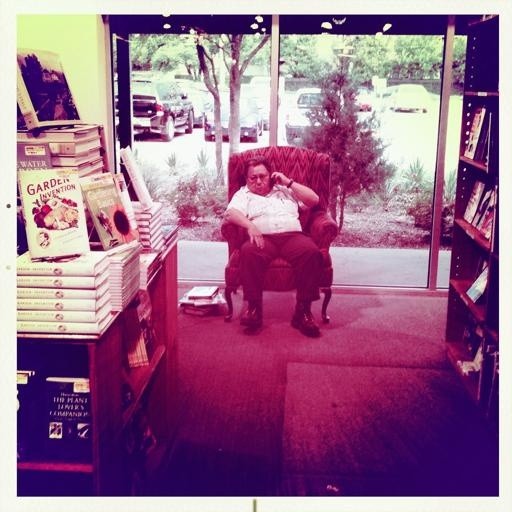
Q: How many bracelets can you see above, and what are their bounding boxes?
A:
[287,179,293,188]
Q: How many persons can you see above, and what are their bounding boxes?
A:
[223,158,322,336]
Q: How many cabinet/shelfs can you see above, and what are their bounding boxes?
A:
[17,238,179,497]
[443,13,499,446]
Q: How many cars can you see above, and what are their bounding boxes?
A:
[388,82,432,114]
[344,85,375,128]
[281,82,331,143]
[130,68,287,143]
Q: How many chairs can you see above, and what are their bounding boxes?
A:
[221,145,339,322]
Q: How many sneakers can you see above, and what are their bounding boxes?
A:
[238,302,264,328]
[291,307,321,338]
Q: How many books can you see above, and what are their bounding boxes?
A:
[457,106,498,421]
[178,286,227,316]
